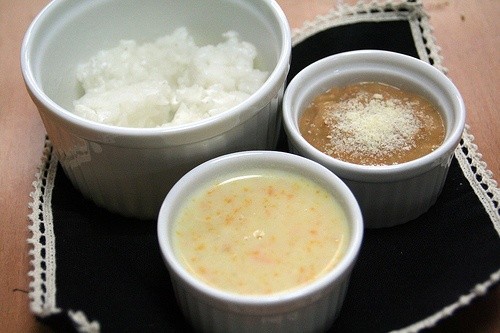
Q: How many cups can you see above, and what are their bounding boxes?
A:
[158,151,364,333]
[282,50,466,226]
[20,0,291,214]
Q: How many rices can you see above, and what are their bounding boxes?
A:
[74,26,269,129]
